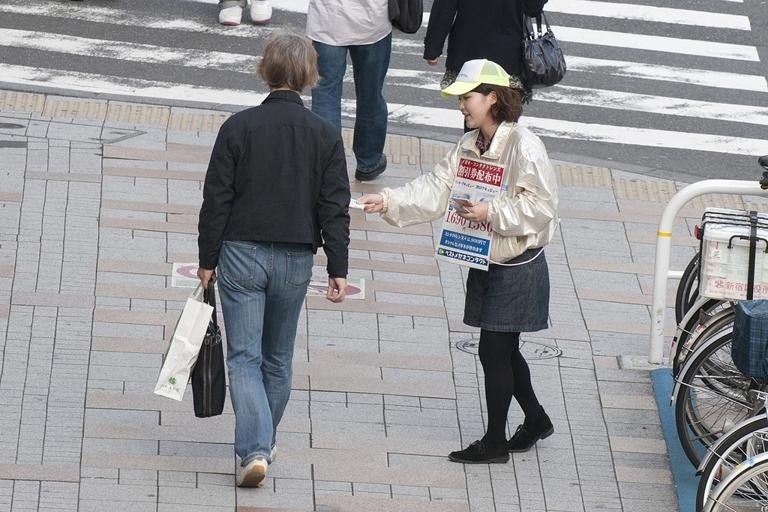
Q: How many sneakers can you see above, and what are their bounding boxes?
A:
[357,155,387,181]
[509,406,553,453]
[448,440,509,464]
[219,6,243,25]
[236,458,267,488]
[250,0,272,22]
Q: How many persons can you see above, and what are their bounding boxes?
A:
[196,34,350,487]
[305,1,393,180]
[358,59,554,464]
[218,1,273,25]
[424,0,549,137]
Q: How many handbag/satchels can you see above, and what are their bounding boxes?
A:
[192,323,226,418]
[389,1,423,35]
[520,29,566,84]
[155,283,214,401]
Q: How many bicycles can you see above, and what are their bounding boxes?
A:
[667,151,767,512]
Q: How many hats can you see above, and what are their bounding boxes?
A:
[441,57,510,99]
